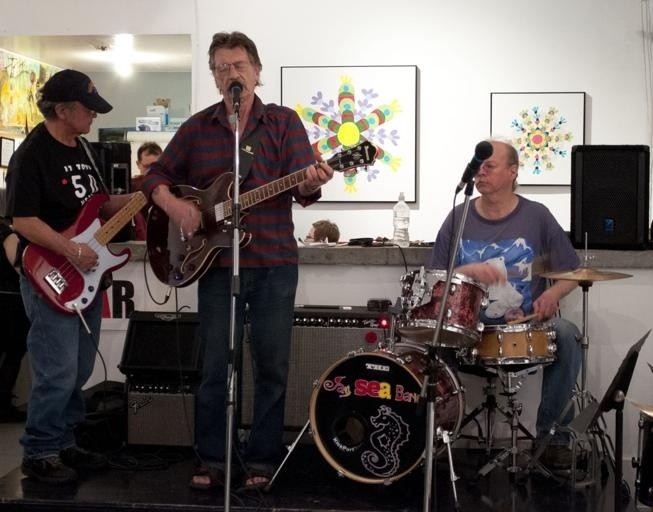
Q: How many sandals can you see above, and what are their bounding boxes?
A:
[189,463,226,490]
[244,461,281,492]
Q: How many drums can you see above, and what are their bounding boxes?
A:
[478,321,557,363]
[309,343,465,485]
[399,265,490,347]
[22,188,146,315]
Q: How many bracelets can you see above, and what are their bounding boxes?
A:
[74,240,83,266]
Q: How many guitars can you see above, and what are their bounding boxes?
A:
[145,138,378,289]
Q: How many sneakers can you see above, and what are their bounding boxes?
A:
[529,442,574,469]
[60,446,104,466]
[21,457,78,483]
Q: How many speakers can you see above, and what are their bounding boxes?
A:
[571,144,649,250]
[283,298,394,429]
[86,140,133,244]
[117,308,204,385]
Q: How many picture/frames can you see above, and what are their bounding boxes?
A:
[280,64,419,204]
[489,91,587,187]
[0,51,65,131]
[0,135,16,168]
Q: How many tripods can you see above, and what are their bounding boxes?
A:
[516,292,614,480]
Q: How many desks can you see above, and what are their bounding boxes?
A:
[84,241,653,452]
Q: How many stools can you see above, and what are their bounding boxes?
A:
[459,365,537,458]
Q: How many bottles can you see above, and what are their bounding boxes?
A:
[393,192,410,248]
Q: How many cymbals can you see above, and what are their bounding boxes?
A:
[626,396,652,420]
[538,267,636,283]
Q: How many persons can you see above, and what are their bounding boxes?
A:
[430,141,585,471]
[304,220,340,244]
[0,215,27,425]
[6,70,148,488]
[141,29,334,494]
[133,142,166,240]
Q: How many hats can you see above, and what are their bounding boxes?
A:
[40,85,113,116]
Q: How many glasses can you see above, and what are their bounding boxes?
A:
[66,104,96,119]
[139,161,152,170]
[211,61,256,76]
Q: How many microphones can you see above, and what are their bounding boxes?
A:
[227,81,245,106]
[361,240,396,247]
[451,140,493,183]
